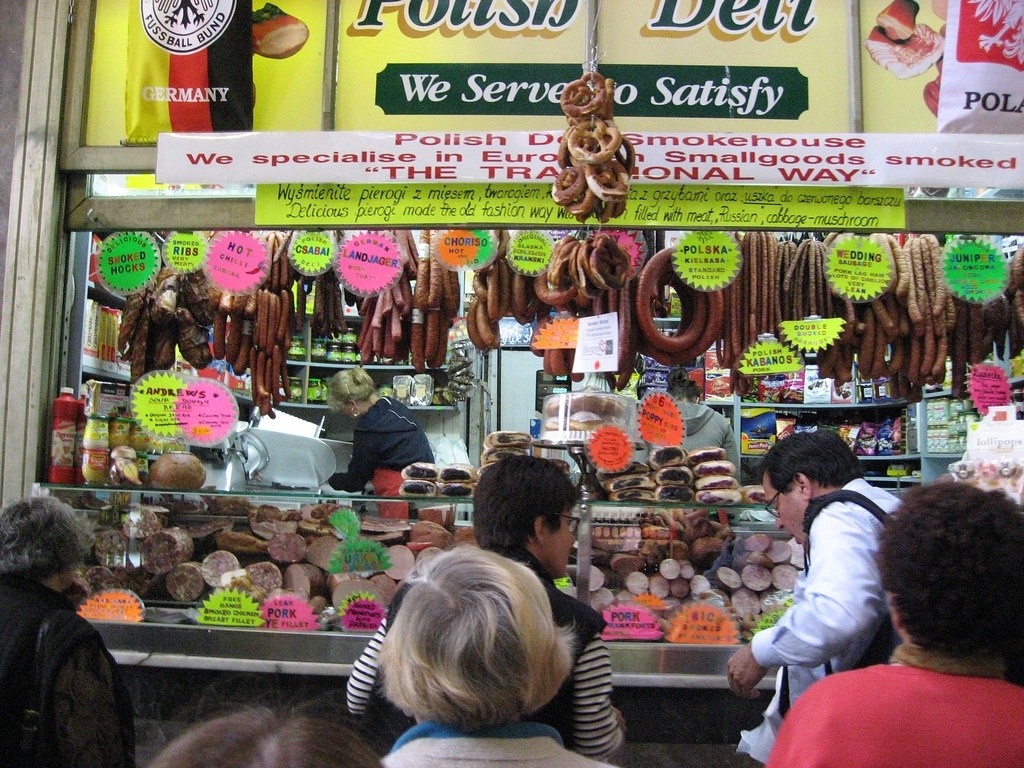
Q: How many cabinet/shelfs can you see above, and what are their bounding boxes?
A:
[608,317,1024,496]
[67,192,462,413]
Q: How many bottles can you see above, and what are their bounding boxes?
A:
[47,384,86,485]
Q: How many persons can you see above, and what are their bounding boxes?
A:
[0,494,135,768]
[763,482,1024,767]
[647,365,741,475]
[143,453,629,768]
[727,430,904,767]
[327,368,435,492]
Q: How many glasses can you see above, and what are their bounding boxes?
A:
[765,484,784,518]
[554,512,580,533]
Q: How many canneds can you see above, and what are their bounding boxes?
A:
[81,415,185,485]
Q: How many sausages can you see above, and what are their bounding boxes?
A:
[114,76,1024,404]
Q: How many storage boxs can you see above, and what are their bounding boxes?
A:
[643,370,671,387]
[643,355,671,370]
[705,369,733,402]
[856,385,872,404]
[681,367,704,400]
[853,354,858,367]
[885,344,893,362]
[741,408,776,454]
[641,387,669,402]
[873,377,892,382]
[776,418,796,442]
[886,464,916,476]
[672,354,704,370]
[855,367,872,386]
[704,338,724,369]
[873,382,895,403]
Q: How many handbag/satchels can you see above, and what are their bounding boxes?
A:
[803,491,902,677]
